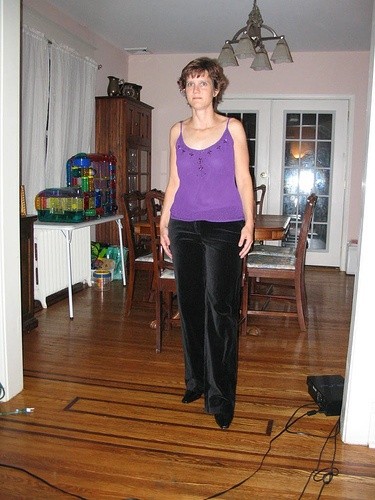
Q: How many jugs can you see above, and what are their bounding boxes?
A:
[107,76,124,97]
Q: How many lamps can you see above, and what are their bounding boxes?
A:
[217,0,293,71]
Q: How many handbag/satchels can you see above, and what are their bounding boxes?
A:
[91,241,128,280]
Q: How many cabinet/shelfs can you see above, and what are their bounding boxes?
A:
[20,214,39,334]
[95,96,154,274]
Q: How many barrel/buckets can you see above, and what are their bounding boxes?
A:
[93,270,112,292]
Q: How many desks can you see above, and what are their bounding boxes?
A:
[33,215,126,320]
[133,215,291,320]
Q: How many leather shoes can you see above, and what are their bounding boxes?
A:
[182,390,201,403]
[215,414,232,428]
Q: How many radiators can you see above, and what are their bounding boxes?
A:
[33,226,93,309]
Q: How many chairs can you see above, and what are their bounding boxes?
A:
[120,185,318,353]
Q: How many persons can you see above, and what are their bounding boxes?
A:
[160,57,255,428]
